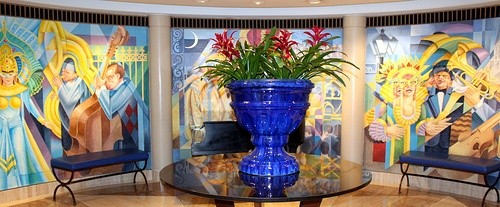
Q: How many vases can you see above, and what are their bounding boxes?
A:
[224,79,315,189]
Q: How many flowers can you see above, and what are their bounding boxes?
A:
[194,25,361,88]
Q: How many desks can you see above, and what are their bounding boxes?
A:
[159,152,372,207]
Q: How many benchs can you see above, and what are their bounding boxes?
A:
[398,151,500,207]
[50,148,149,206]
[190,120,305,157]
